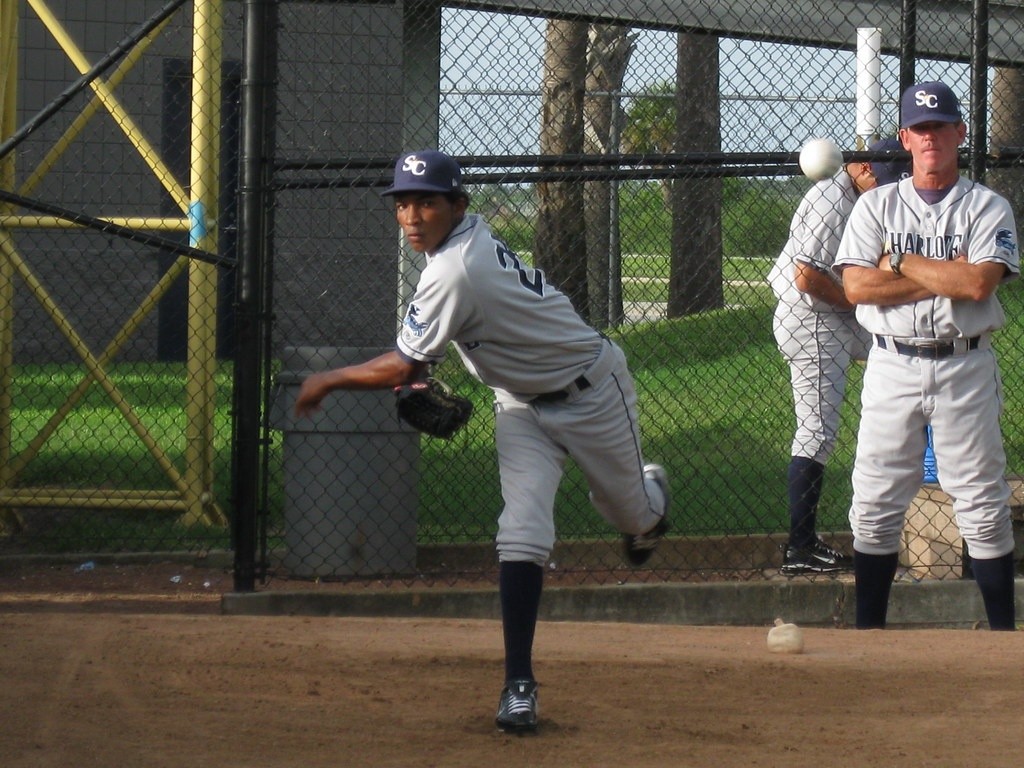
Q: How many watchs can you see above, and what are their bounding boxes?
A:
[889,252,905,275]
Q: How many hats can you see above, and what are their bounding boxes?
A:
[866,139,913,187]
[379,150,463,196]
[900,81,961,128]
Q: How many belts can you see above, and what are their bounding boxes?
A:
[875,334,980,359]
[527,332,613,407]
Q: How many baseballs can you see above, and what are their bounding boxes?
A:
[798,137,844,182]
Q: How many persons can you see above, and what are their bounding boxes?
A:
[831,80,1020,631]
[767,138,914,578]
[295,152,672,725]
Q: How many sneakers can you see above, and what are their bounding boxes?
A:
[623,464,669,565]
[778,536,855,577]
[495,679,539,731]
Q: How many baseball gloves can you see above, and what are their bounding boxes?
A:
[392,377,475,442]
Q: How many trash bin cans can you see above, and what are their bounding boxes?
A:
[260,345,424,579]
